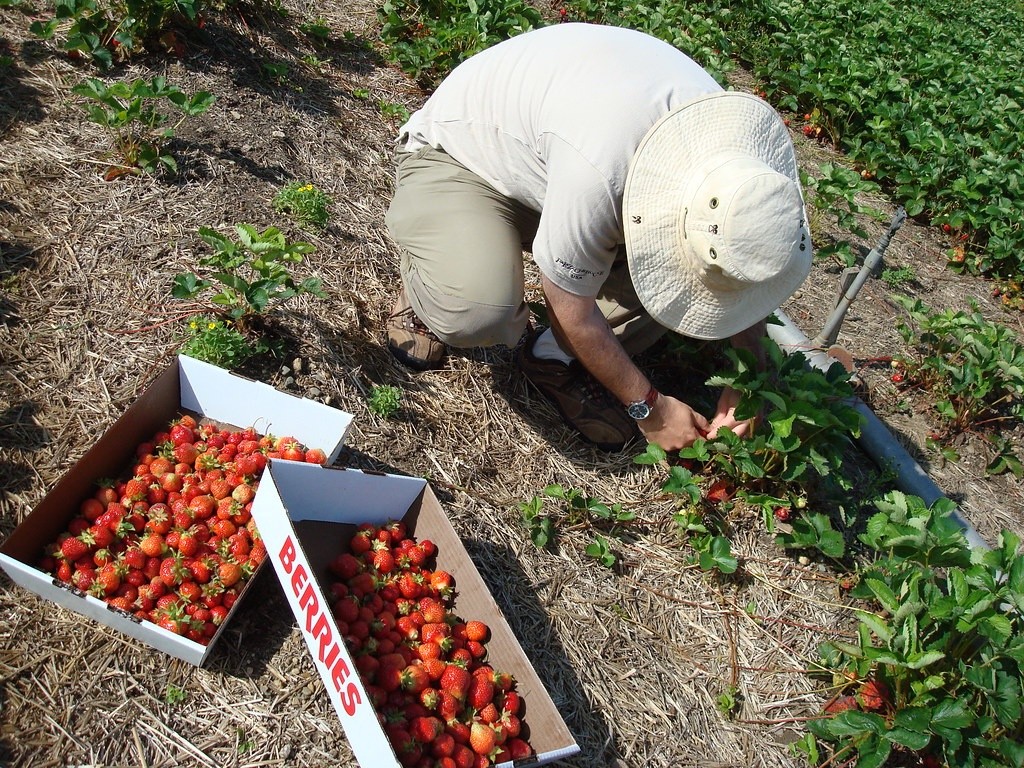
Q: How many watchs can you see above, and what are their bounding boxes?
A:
[623,384,659,420]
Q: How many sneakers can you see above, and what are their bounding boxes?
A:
[385,285,447,371]
[517,322,640,453]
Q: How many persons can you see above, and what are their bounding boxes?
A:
[384,21,812,454]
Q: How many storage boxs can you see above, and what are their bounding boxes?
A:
[251,460,581,768]
[0,351,356,666]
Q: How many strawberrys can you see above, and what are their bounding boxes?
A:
[0,0,1024,768]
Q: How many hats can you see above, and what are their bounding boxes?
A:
[622,92,813,340]
